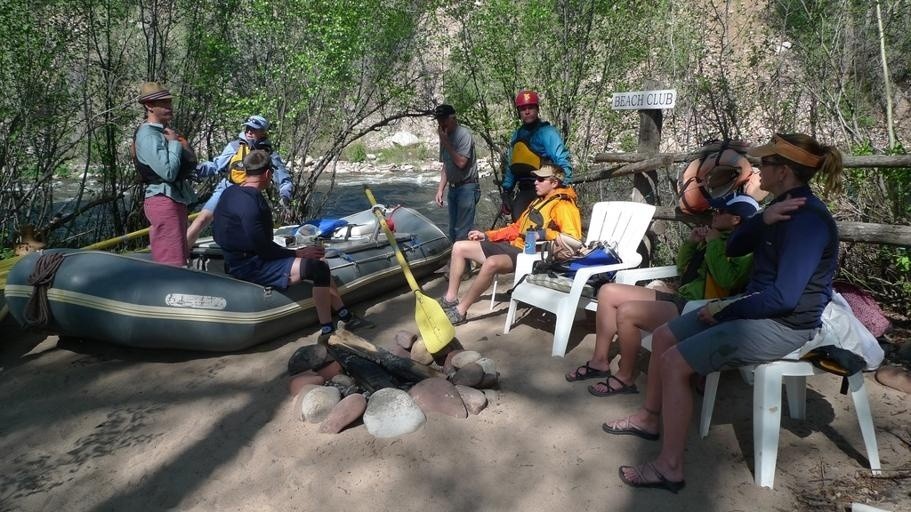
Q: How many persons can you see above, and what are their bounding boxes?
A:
[133,81,197,268]
[186,114,296,255]
[211,149,376,346]
[435,162,582,326]
[502,91,574,226]
[432,104,481,281]
[565,196,762,396]
[602,131,844,492]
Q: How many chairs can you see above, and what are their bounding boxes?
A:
[503,200,656,358]
[610,262,720,354]
[489,220,556,325]
[698,284,885,489]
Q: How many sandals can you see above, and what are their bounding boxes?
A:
[589,375,640,397]
[601,416,663,440]
[617,463,689,493]
[563,361,614,380]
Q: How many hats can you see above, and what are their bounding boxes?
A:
[710,193,763,218]
[514,90,541,107]
[748,135,828,171]
[241,116,268,130]
[529,164,567,178]
[138,81,176,104]
[431,104,456,121]
[243,149,279,176]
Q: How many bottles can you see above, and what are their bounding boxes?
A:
[525,224,537,253]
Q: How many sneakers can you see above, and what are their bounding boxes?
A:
[447,308,474,327]
[434,296,461,307]
[344,311,377,333]
[316,332,334,346]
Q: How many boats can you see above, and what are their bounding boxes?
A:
[5,204,451,354]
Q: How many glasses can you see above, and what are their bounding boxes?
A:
[711,207,726,214]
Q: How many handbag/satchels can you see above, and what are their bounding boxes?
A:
[165,125,197,181]
[804,342,869,395]
[228,144,248,185]
